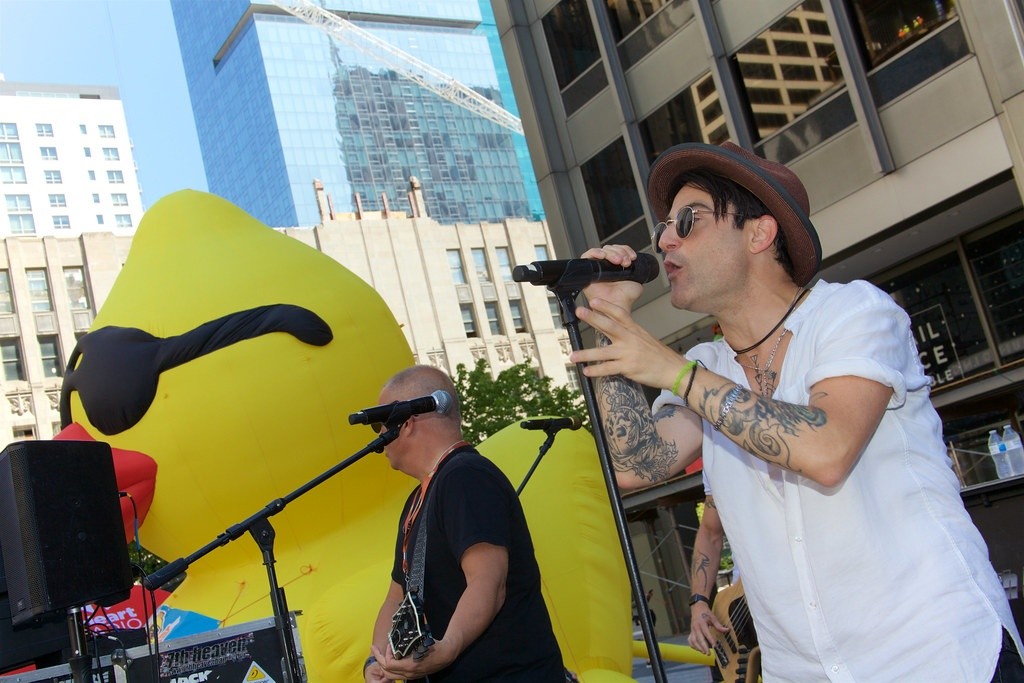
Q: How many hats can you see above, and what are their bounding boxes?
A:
[647,142,822,286]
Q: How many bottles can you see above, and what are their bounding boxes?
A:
[1001,425,1024,475]
[988,430,1010,479]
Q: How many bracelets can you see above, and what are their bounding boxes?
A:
[672,359,708,406]
[363,656,378,679]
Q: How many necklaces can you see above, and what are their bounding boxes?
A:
[733,288,811,396]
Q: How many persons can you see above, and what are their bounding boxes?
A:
[569,142,1024,683]
[687,469,740,655]
[362,364,569,683]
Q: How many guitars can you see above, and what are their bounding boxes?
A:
[710,576,760,683]
[388,586,435,661]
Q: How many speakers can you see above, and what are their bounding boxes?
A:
[0,439,133,672]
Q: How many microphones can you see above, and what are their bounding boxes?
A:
[513,252,660,284]
[521,416,582,430]
[349,389,453,425]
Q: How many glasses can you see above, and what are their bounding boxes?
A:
[650,207,760,253]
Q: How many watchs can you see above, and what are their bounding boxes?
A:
[689,594,712,608]
